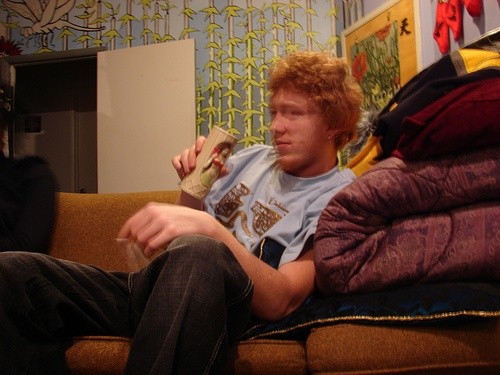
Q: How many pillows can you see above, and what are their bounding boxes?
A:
[239,277,500,340]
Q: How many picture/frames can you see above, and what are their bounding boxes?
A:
[340,0,422,114]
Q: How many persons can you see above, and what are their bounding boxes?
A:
[1,49,364,375]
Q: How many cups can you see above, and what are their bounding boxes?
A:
[181,123,238,201]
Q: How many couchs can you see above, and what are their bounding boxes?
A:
[50,27,500,375]
